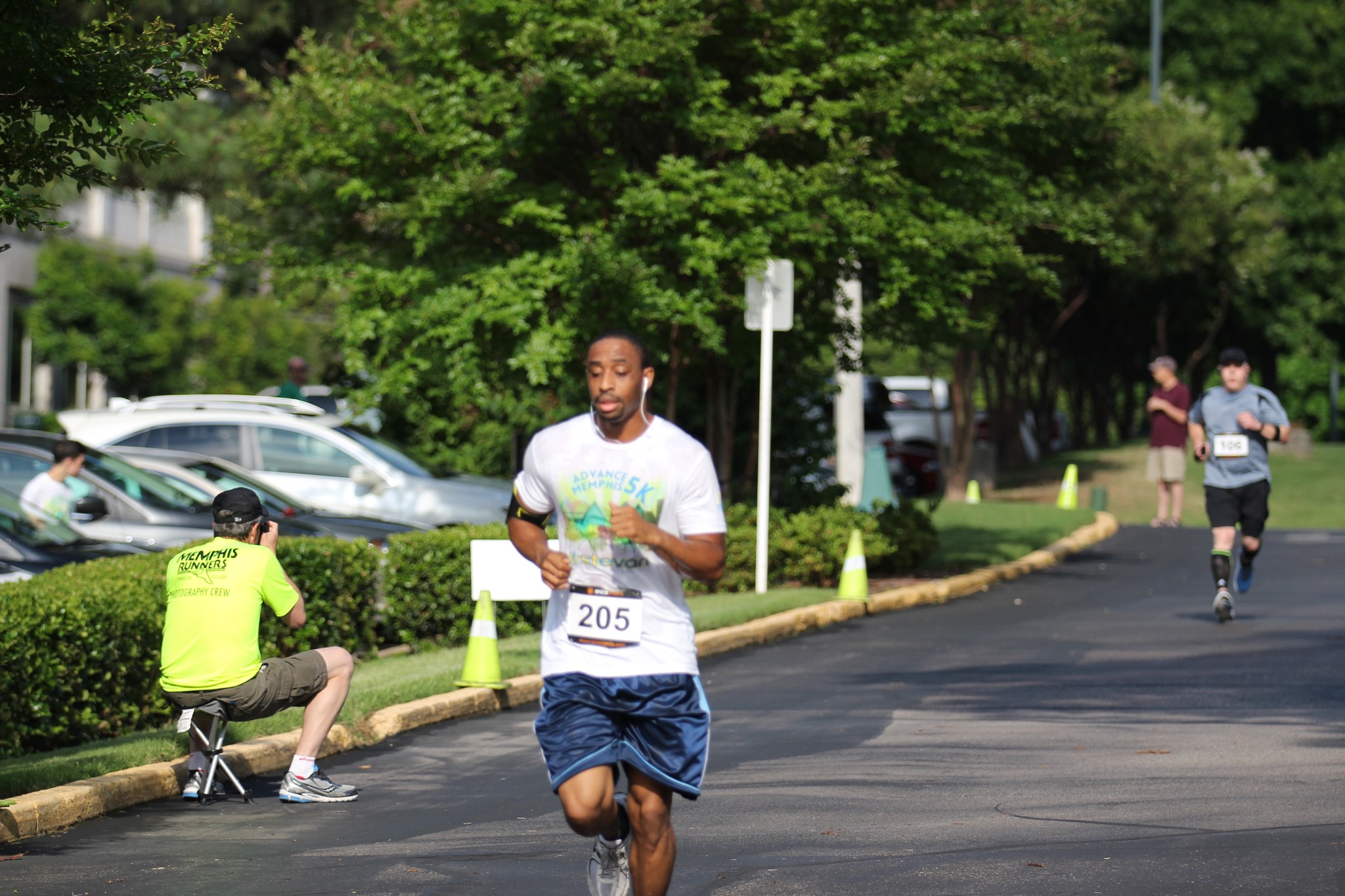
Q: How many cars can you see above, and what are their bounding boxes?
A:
[0,488,166,584]
[880,374,955,498]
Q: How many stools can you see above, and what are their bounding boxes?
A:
[177,700,256,806]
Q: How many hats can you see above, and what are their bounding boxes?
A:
[1147,355,1176,372]
[1217,347,1246,366]
[213,487,270,525]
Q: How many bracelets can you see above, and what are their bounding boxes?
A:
[1258,423,1264,433]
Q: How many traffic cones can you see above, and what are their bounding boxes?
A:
[1054,463,1079,511]
[964,480,982,505]
[830,528,873,604]
[452,590,513,690]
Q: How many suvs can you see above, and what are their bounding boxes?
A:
[0,383,559,551]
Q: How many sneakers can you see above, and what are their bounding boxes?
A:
[279,764,358,802]
[182,768,228,799]
[587,790,632,896]
[1211,589,1236,622]
[1233,544,1254,594]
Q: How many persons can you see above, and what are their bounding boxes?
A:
[1188,347,1291,619]
[272,359,313,453]
[158,487,359,802]
[1147,357,1192,527]
[862,377,900,513]
[19,441,87,543]
[505,327,727,896]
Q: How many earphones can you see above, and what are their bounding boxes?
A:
[643,377,649,391]
